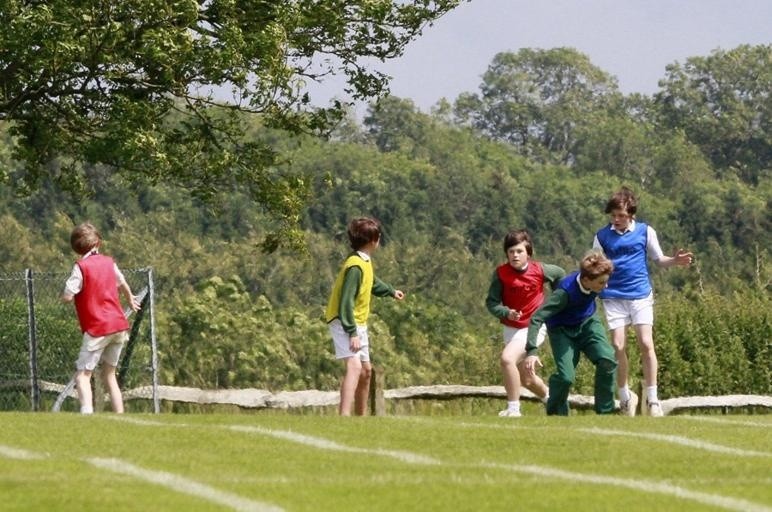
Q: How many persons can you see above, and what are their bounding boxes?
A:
[486,230,567,416]
[593,193,694,419]
[524,251,619,416]
[61,221,142,414]
[323,216,405,417]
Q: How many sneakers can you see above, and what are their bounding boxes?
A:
[646,395,665,417]
[620,391,639,416]
[496,409,522,417]
